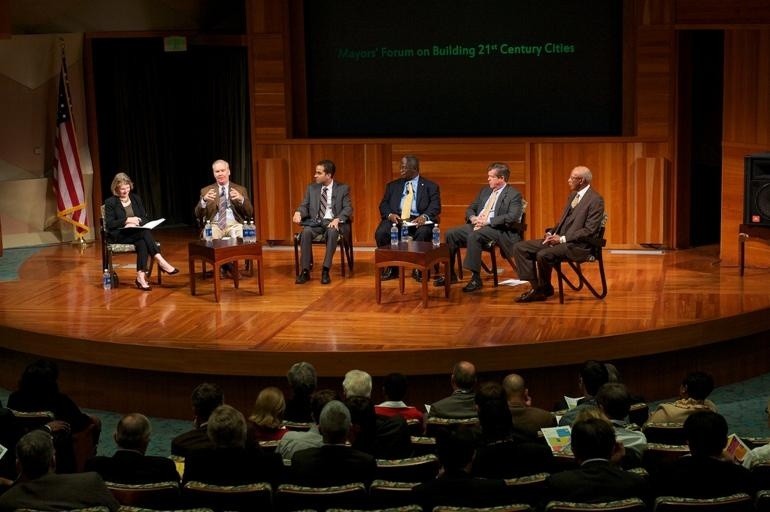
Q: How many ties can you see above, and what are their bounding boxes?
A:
[216,186,228,232]
[571,194,580,209]
[311,187,329,225]
[473,191,498,231]
[400,182,414,229]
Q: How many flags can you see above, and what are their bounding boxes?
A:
[51,47,89,239]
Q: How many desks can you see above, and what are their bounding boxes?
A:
[188,240,264,303]
[375,241,452,307]
[740,223,770,276]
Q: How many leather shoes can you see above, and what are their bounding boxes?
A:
[412,268,423,282]
[294,270,310,284]
[462,277,483,292]
[321,270,330,284]
[514,285,555,303]
[221,265,242,281]
[433,273,458,287]
[380,266,399,281]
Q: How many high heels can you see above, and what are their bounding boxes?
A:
[158,261,179,275]
[135,278,152,291]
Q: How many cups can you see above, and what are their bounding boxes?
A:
[230,228,236,238]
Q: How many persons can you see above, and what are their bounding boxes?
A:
[292,159,354,284]
[375,155,441,282]
[433,162,522,292]
[195,159,254,280]
[513,166,604,302]
[0,356,769,511]
[105,172,179,291]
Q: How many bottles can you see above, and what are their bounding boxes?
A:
[391,220,408,246]
[205,220,214,243]
[432,224,440,248]
[243,220,256,243]
[102,269,119,289]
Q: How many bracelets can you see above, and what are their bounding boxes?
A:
[388,213,392,220]
[203,197,206,202]
[420,215,427,220]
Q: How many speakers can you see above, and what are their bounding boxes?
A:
[743,154,770,226]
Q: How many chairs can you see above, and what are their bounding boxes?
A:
[99,204,162,284]
[195,216,253,280]
[293,219,354,276]
[545,212,608,303]
[456,200,527,287]
